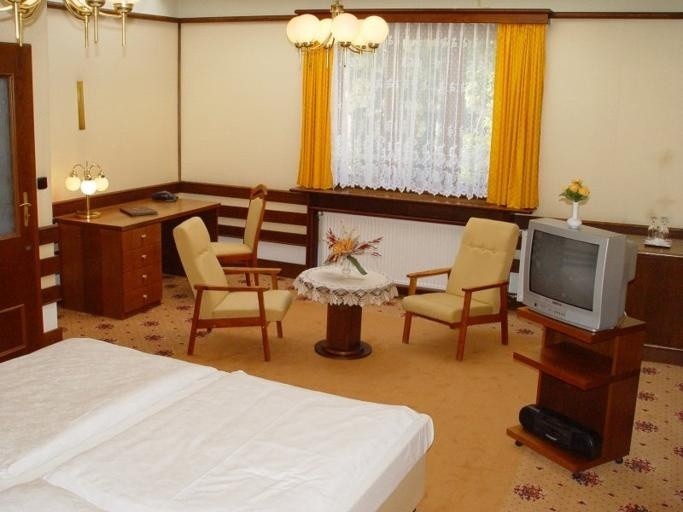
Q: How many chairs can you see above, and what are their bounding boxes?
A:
[398,216,521,363]
[208,184,270,287]
[172,215,295,362]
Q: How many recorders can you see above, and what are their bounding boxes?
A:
[519,403,601,460]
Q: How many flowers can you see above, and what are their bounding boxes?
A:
[557,178,591,202]
[320,224,384,279]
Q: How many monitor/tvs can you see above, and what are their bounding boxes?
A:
[517,218,638,333]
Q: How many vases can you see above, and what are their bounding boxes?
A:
[340,257,351,279]
[566,201,582,229]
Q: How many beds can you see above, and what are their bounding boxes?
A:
[0,335,435,511]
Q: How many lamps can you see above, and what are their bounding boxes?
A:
[64,159,109,219]
[0,0,133,49]
[282,0,390,68]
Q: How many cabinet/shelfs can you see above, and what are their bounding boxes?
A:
[505,305,648,473]
[616,234,683,370]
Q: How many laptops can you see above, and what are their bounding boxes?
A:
[120,206,158,217]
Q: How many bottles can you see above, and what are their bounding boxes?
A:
[646,215,669,247]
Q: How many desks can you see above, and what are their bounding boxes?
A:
[53,193,220,320]
[292,265,400,362]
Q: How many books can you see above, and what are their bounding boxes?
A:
[119,205,159,217]
